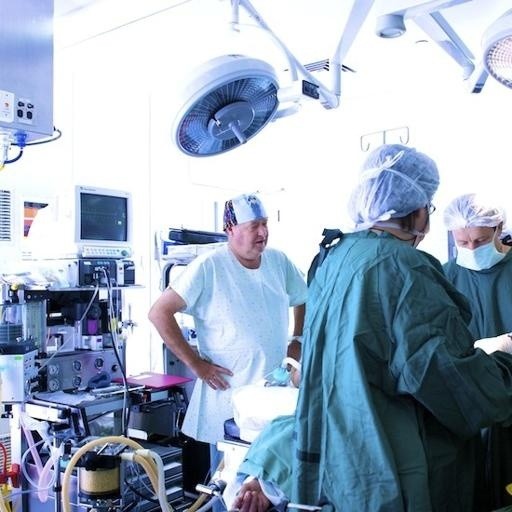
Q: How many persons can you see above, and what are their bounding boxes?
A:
[442,191,512,357]
[147,193,310,511]
[231,479,272,512]
[284,143,511,512]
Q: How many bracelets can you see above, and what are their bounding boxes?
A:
[288,334,303,343]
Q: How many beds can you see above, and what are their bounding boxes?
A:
[216,387,299,479]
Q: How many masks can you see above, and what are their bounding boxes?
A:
[456,240,507,273]
[413,216,430,248]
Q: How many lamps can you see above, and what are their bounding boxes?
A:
[375,1,512,93]
[168,0,374,157]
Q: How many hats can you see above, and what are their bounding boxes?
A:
[224,194,268,232]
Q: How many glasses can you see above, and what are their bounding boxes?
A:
[430,203,435,214]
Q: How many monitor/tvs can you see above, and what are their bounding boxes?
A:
[74,185,136,259]
